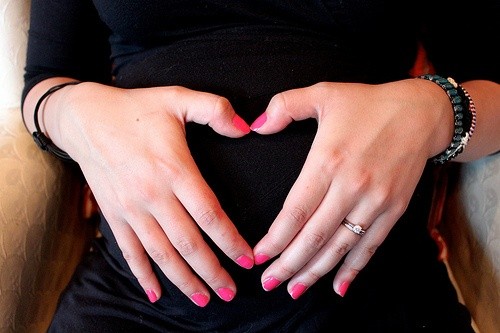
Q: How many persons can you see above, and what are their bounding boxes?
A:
[19,0,500,332]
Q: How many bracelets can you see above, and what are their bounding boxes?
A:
[415,74,476,165]
[31,81,81,160]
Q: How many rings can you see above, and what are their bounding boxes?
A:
[341,219,367,237]
